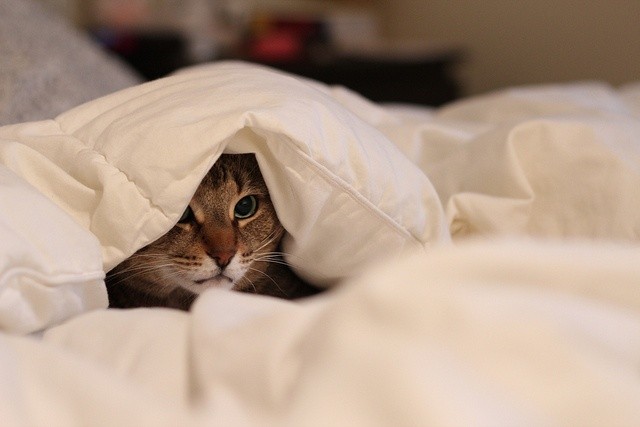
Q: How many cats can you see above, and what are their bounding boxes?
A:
[106,154,326,312]
